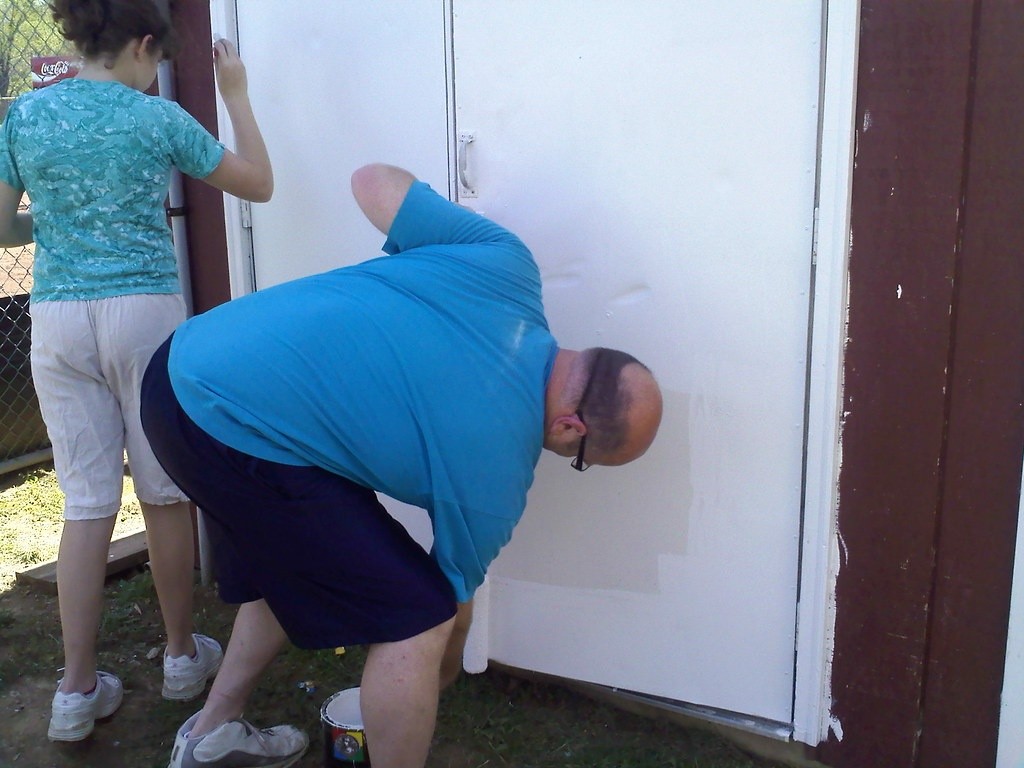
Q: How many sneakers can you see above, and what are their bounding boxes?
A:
[168,709,309,768]
[162,634,223,700]
[48,664,124,741]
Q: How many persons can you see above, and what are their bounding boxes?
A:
[141,163,663,768]
[0,0,274,741]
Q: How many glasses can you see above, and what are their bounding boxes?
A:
[571,409,591,471]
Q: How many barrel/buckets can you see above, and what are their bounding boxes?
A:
[320,687,372,768]
